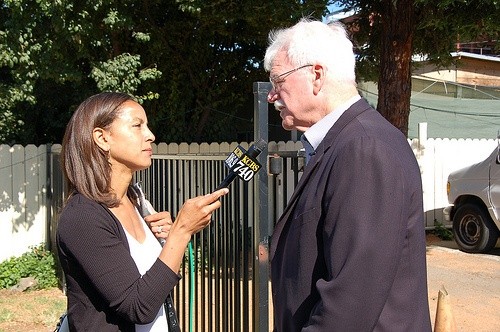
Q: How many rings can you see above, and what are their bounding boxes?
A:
[157,226,162,234]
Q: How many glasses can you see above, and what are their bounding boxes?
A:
[267,64,314,94]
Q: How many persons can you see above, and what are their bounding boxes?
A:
[264,18,433,332]
[53,91,230,331]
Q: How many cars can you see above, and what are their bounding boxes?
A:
[445,145,497,254]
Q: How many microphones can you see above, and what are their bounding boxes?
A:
[208,138,267,205]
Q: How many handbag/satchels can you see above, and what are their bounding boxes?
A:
[53,312,70,332]
